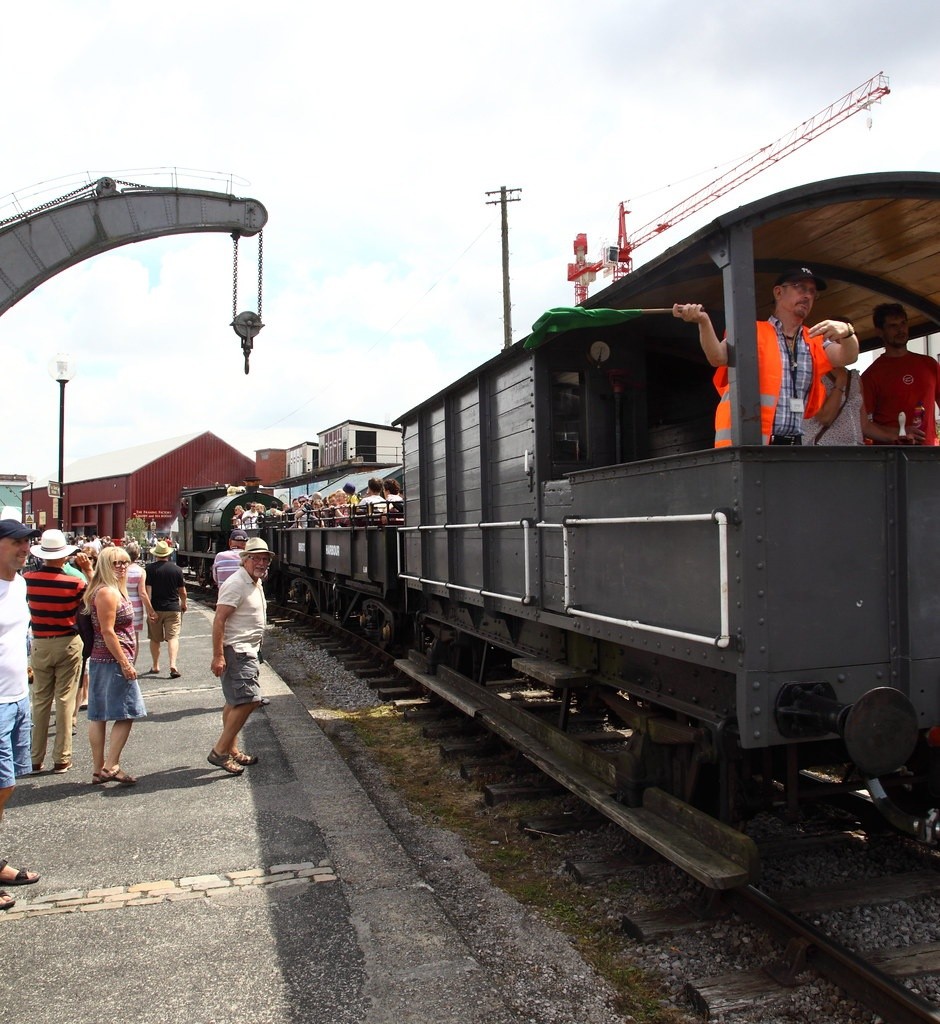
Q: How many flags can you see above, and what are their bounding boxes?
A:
[523,306,644,348]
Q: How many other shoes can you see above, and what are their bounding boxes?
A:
[29,763,44,774]
[55,761,72,773]
[72,723,78,735]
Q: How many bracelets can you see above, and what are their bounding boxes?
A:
[832,386,845,393]
[150,611,156,617]
[120,664,132,671]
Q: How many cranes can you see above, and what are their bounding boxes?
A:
[568,71,890,302]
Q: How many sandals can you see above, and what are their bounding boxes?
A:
[207,748,258,773]
[92,768,138,785]
[0,859,40,909]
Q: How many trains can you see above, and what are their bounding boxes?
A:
[176,167,940,881]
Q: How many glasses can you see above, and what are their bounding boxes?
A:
[885,318,912,330]
[247,555,272,563]
[299,501,305,503]
[111,560,130,568]
[779,284,821,300]
[88,557,95,560]
[119,541,126,543]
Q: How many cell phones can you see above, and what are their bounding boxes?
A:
[68,548,82,556]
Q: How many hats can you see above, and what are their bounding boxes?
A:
[239,538,275,557]
[0,519,42,540]
[30,529,79,559]
[149,541,174,558]
[343,483,355,493]
[778,268,827,292]
[229,530,248,541]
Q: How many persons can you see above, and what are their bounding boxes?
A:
[124,545,160,668]
[26,533,179,573]
[0,519,40,914]
[80,546,148,785]
[211,537,275,775]
[22,529,98,773]
[673,265,940,448]
[213,530,271,707]
[230,478,403,528]
[143,541,188,677]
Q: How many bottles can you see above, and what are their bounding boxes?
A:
[913,401,925,445]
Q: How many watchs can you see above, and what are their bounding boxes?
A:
[844,321,855,338]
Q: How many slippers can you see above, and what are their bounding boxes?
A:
[149,667,160,674]
[170,670,181,677]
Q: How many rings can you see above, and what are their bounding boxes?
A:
[212,669,216,672]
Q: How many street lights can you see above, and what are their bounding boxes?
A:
[27,474,36,512]
[54,350,71,530]
